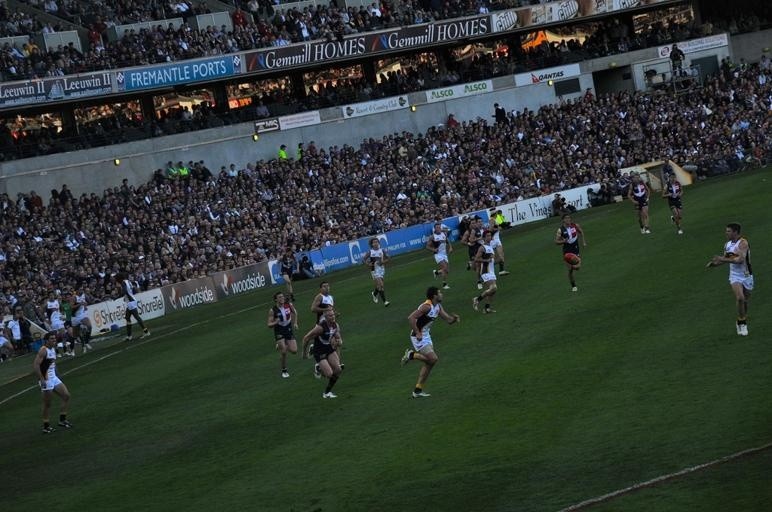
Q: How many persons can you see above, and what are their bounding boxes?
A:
[526,46,772,216]
[553,212,587,291]
[661,173,684,235]
[400,287,461,399]
[626,174,652,235]
[32,331,74,435]
[0,104,524,361]
[705,223,754,336]
[267,291,300,380]
[524,7,771,71]
[300,305,345,399]
[1,0,526,161]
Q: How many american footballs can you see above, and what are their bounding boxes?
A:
[565,253,580,265]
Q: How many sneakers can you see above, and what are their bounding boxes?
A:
[272,344,349,403]
[441,283,450,290]
[410,390,432,398]
[399,346,413,368]
[57,342,92,360]
[498,270,511,276]
[570,285,578,292]
[369,291,390,307]
[121,327,151,343]
[432,269,438,280]
[40,419,74,434]
[472,280,498,315]
[640,228,651,235]
[466,260,473,271]
[734,318,750,336]
[670,215,684,235]
